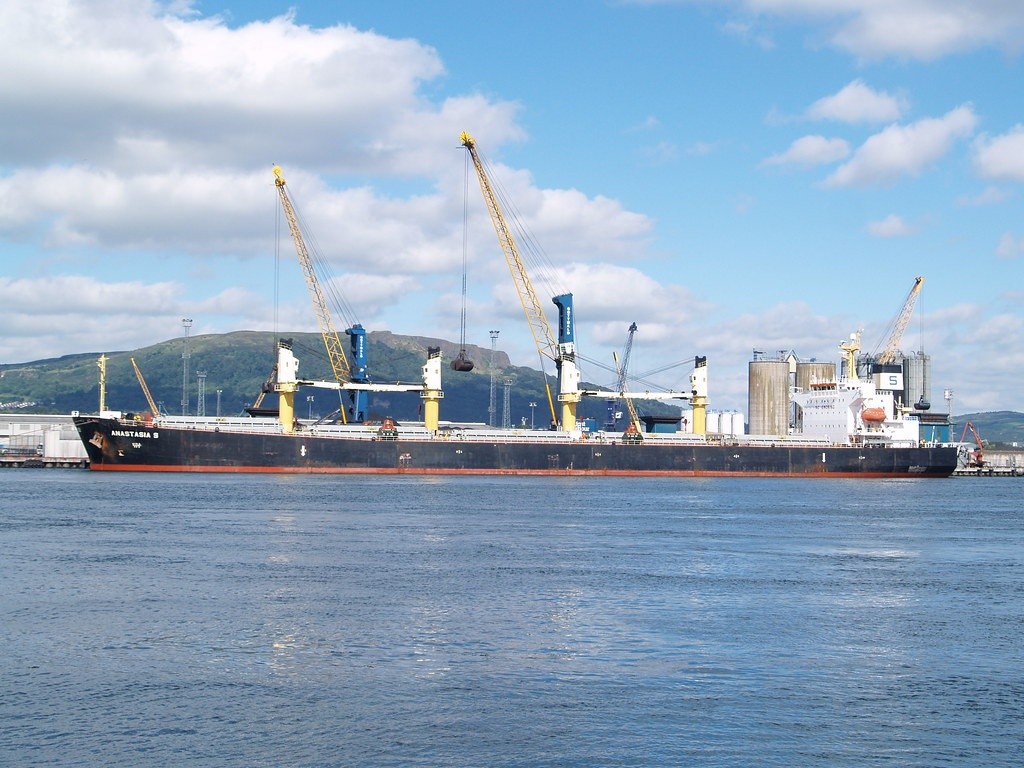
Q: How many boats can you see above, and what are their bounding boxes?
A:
[69,130,960,478]
